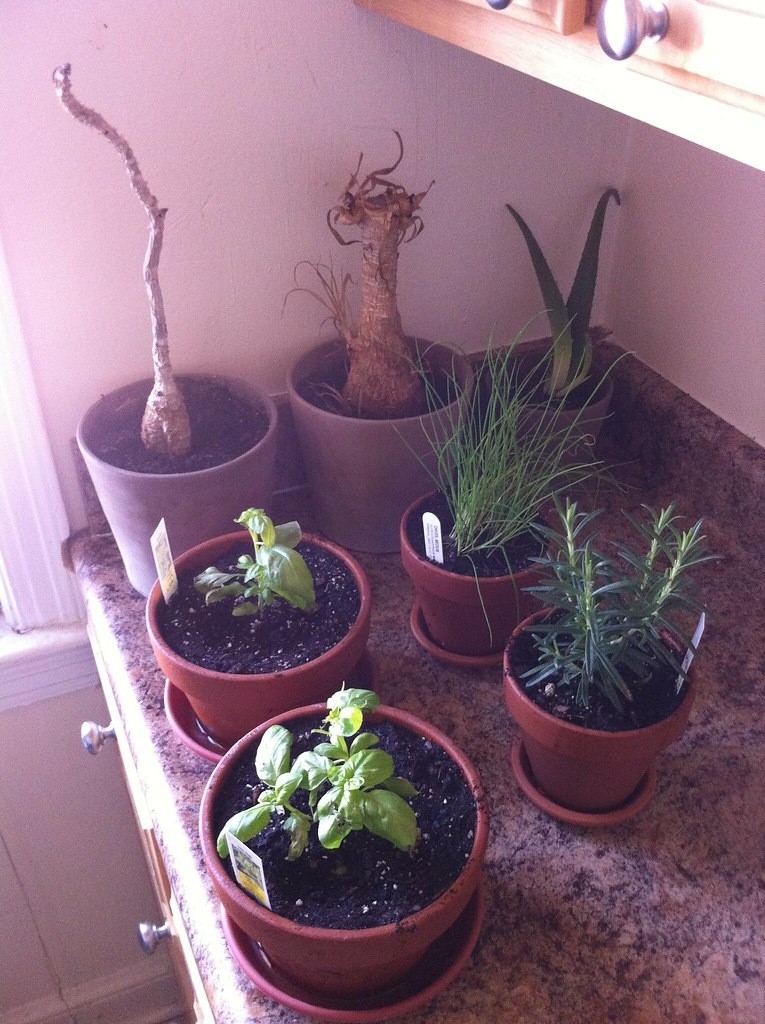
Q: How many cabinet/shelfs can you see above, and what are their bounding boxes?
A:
[350,0,765,176]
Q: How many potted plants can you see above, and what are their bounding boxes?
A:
[200,680,488,1024]
[389,310,636,669]
[490,188,621,496]
[504,492,725,826]
[145,508,372,764]
[50,61,278,599]
[280,128,473,556]
